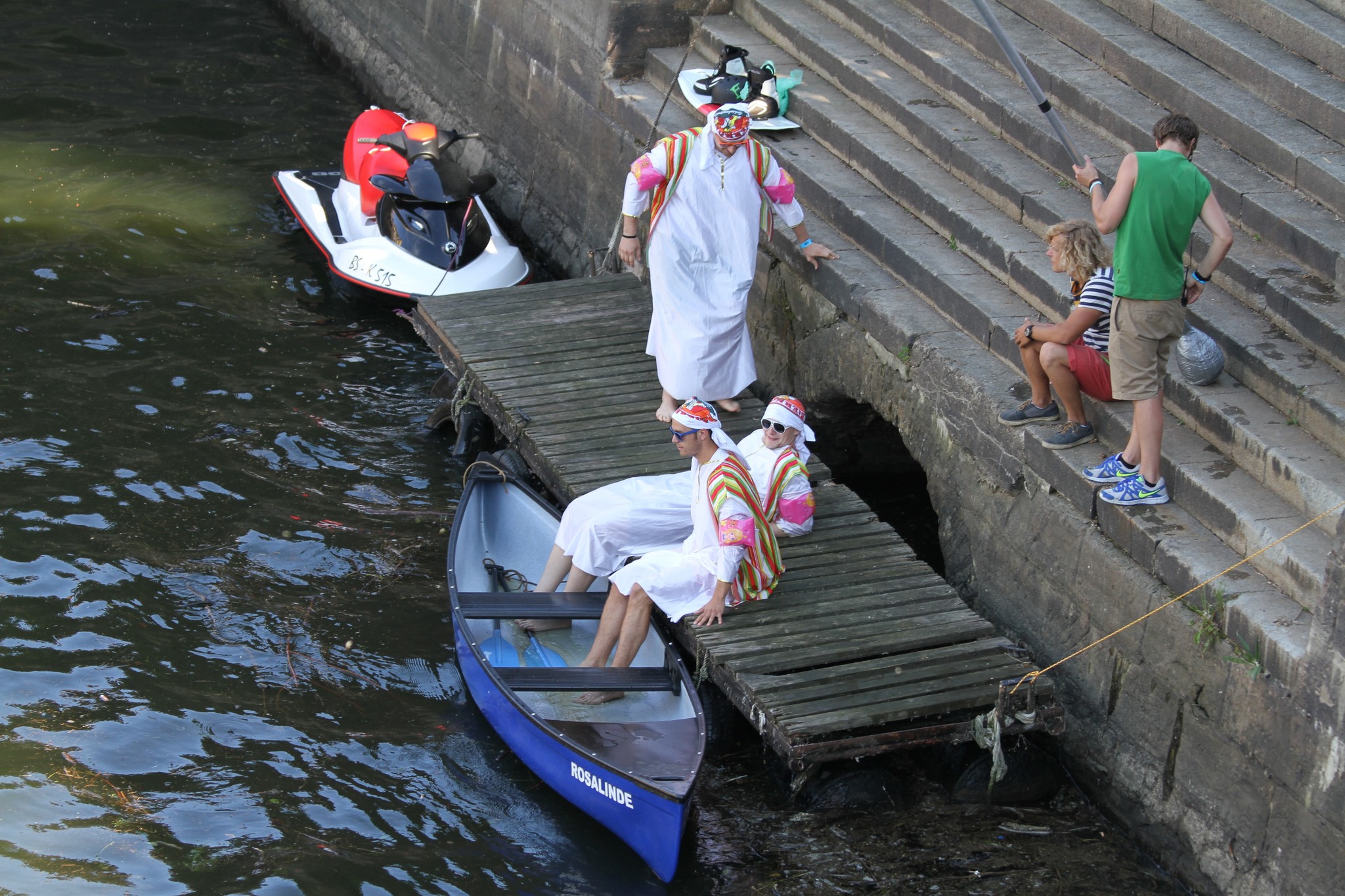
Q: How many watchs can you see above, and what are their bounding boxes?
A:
[1024,325,1035,341]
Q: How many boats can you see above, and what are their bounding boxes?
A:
[446,453,708,881]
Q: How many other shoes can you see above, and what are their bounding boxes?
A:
[748,64,779,109]
[747,93,779,120]
[693,73,730,95]
[716,44,749,79]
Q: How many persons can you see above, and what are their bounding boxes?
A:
[998,217,1120,448]
[572,396,788,705]
[618,103,840,425]
[514,395,816,631]
[1072,113,1234,505]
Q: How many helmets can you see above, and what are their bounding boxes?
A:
[712,75,754,103]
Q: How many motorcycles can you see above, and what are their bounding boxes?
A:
[272,104,536,300]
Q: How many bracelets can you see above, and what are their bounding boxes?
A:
[622,233,636,239]
[1088,178,1103,193]
[800,238,812,249]
[1190,270,1212,285]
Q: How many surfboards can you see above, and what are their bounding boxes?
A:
[677,59,801,132]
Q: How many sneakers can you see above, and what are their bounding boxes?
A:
[1099,474,1170,505]
[1043,419,1095,449]
[998,398,1061,425]
[1082,452,1140,483]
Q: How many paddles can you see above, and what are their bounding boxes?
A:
[481,565,571,667]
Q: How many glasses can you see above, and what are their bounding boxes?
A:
[715,134,748,147]
[1188,146,1194,162]
[761,419,789,434]
[669,426,698,441]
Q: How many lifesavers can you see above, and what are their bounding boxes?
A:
[478,446,526,486]
[419,396,489,461]
[487,445,530,481]
